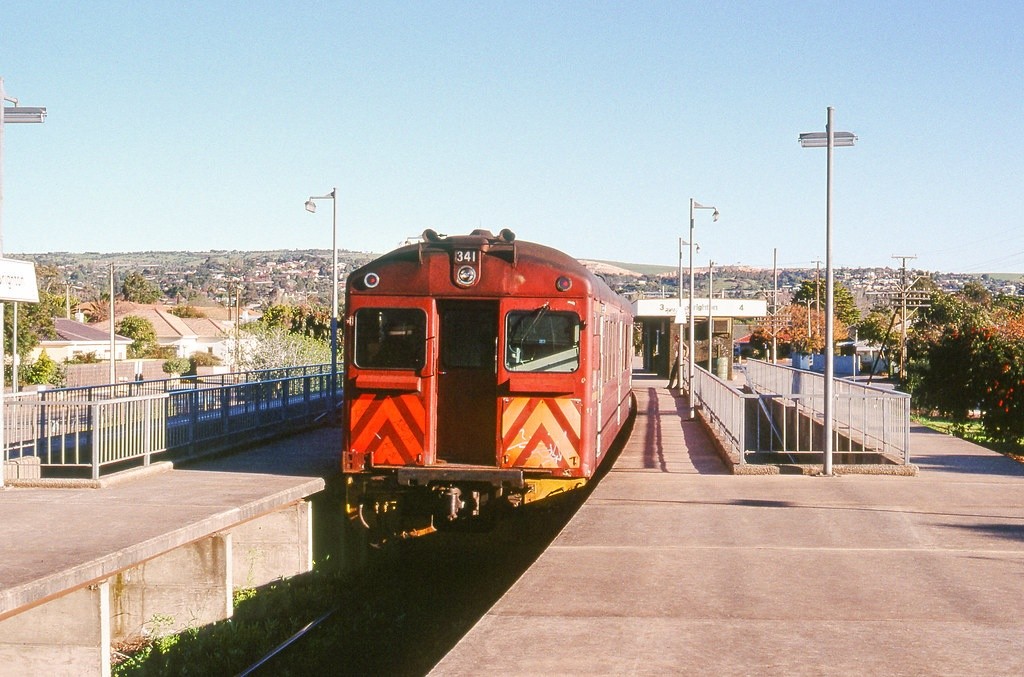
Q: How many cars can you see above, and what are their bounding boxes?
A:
[173,394,217,414]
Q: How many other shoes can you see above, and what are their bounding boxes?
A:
[673,385,678,389]
[664,384,672,389]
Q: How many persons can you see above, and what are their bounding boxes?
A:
[750,330,770,360]
[663,335,689,389]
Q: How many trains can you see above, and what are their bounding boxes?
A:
[342,227,635,583]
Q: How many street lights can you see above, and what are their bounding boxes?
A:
[689,206,719,421]
[1,95,49,489]
[678,242,701,396]
[304,195,337,422]
[799,106,859,475]
[709,263,718,375]
